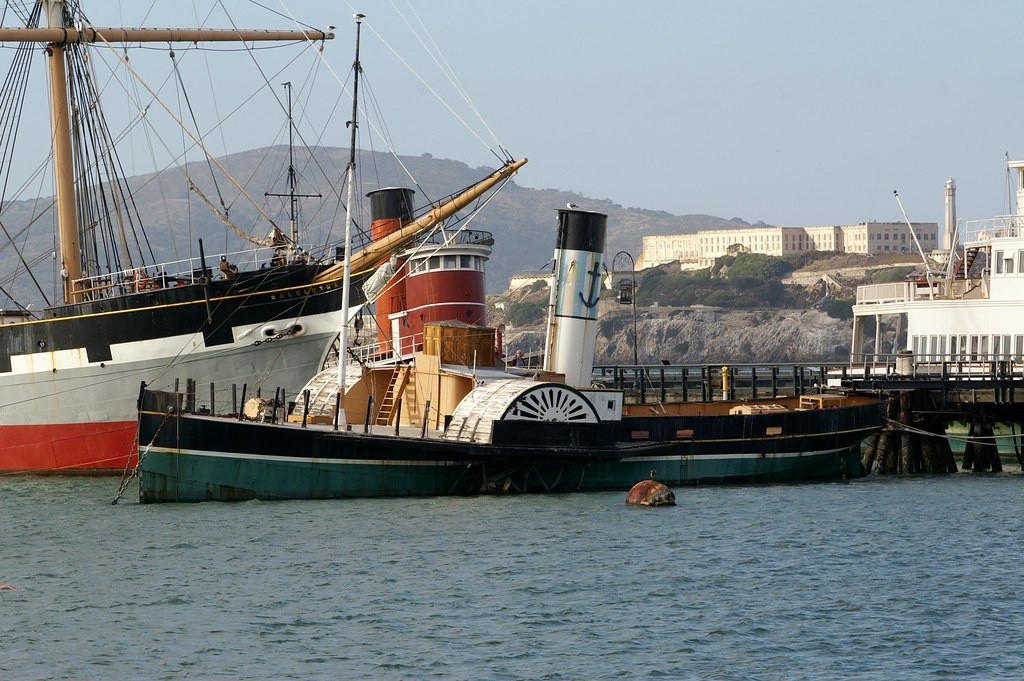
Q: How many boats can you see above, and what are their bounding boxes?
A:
[0,0,530,479]
[826,150,1024,387]
[136,11,893,506]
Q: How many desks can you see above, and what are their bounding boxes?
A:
[800,394,848,410]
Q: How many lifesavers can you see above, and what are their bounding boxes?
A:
[132,270,147,289]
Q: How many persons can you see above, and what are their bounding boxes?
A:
[286,245,298,266]
[273,248,286,266]
[511,350,526,366]
